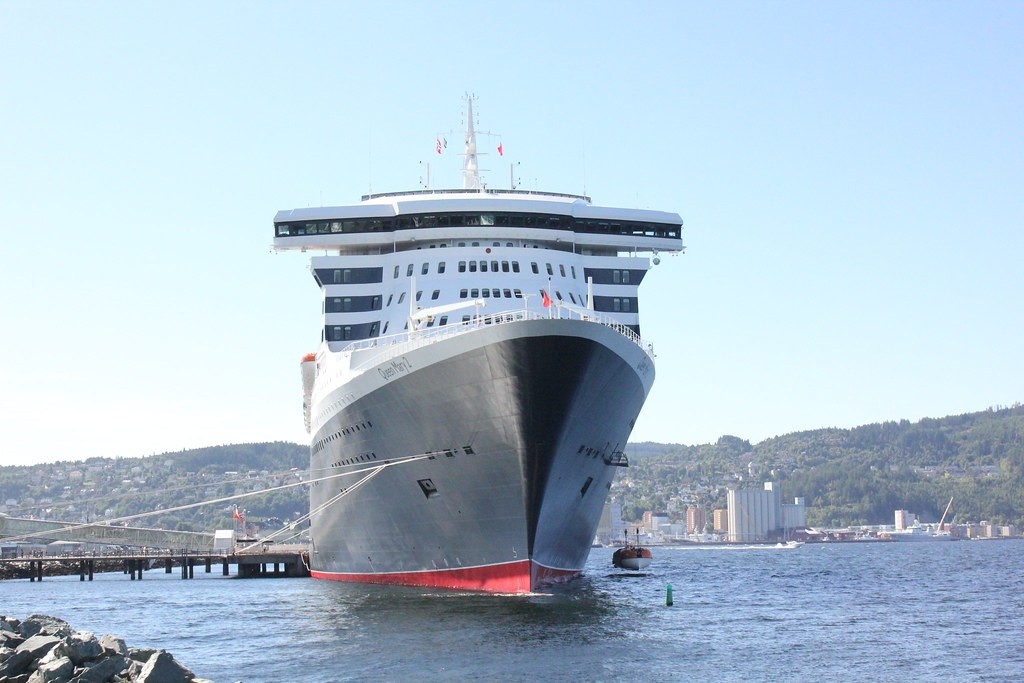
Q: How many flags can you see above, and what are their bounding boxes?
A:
[544,290,553,307]
[444,138,447,149]
[234,507,246,525]
[437,138,441,154]
[498,142,502,155]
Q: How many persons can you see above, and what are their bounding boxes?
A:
[21,548,43,558]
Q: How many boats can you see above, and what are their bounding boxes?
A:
[784,541,805,548]
[612,527,651,570]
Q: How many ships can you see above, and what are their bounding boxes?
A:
[268,91,687,594]
